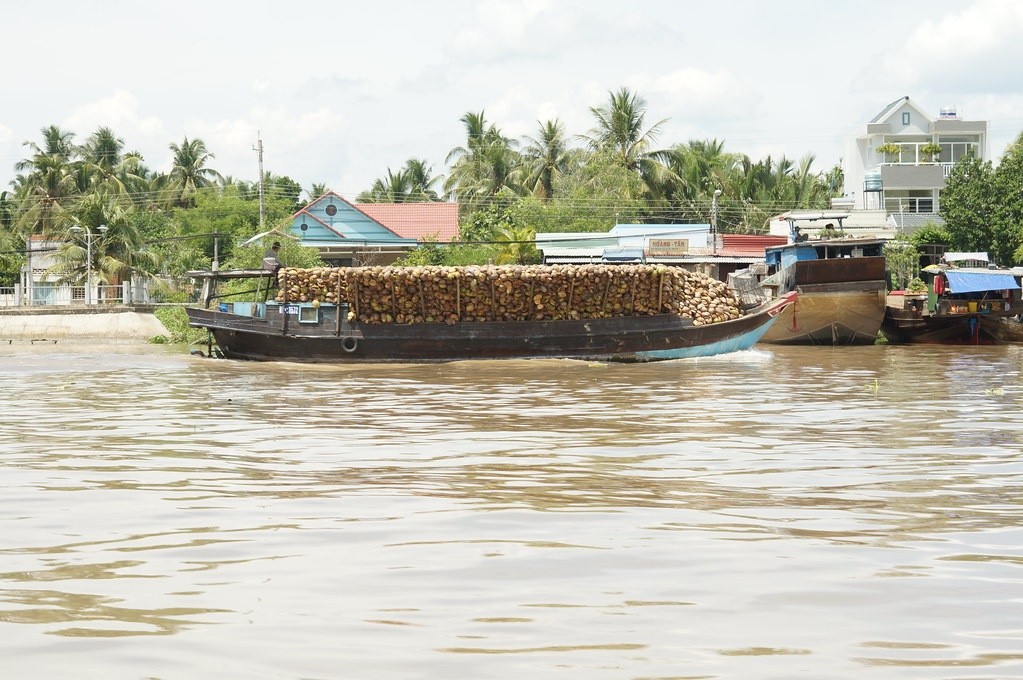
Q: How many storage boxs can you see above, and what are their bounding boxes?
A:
[750,263,769,276]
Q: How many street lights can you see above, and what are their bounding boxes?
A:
[68,224,110,308]
[712,189,722,253]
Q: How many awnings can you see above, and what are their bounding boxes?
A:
[602,248,645,260]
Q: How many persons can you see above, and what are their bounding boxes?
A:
[266,242,284,288]
[826,223,833,229]
[795,226,808,242]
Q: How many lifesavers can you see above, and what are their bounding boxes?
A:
[341,336,358,353]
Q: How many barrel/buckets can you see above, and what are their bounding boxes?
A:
[968,301,977,312]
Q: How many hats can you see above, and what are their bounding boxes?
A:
[795,226,801,231]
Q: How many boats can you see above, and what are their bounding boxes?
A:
[741,212,893,346]
[181,274,798,365]
[878,305,1008,346]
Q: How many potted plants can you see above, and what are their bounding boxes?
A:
[815,229,848,241]
